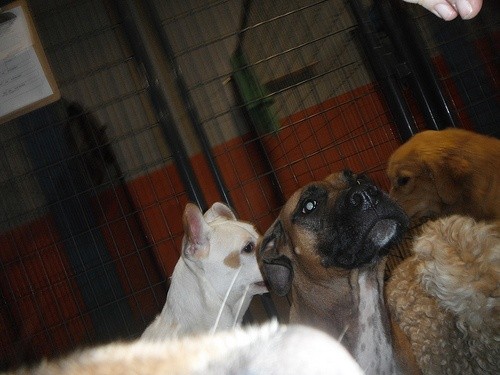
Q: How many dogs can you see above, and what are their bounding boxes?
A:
[255,170,410,375]
[388,128,500,219]
[139,201,270,340]
